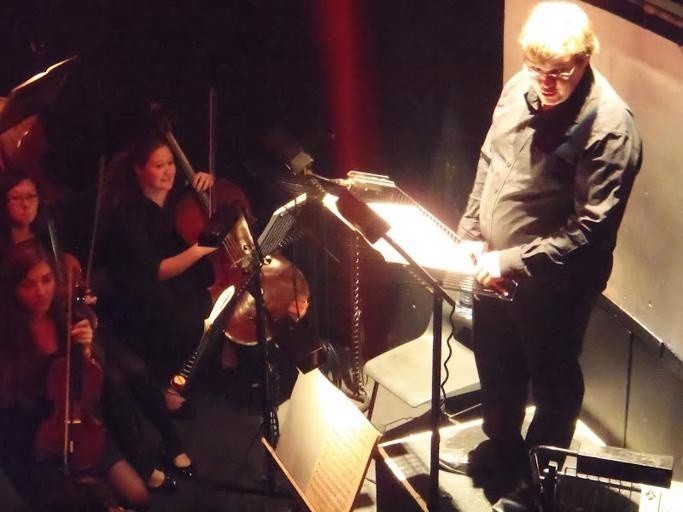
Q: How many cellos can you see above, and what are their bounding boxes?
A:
[149,89,311,345]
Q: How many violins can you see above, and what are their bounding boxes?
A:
[35,205,97,336]
[32,265,108,474]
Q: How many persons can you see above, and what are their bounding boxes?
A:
[1,241,148,510]
[4,170,194,494]
[438,1,643,488]
[99,139,215,363]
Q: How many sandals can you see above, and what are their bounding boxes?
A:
[146,470,178,493]
[173,455,194,480]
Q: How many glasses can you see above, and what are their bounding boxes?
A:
[524,59,579,80]
[6,194,39,203]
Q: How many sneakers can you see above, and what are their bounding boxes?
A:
[439,443,510,477]
[492,481,543,512]
